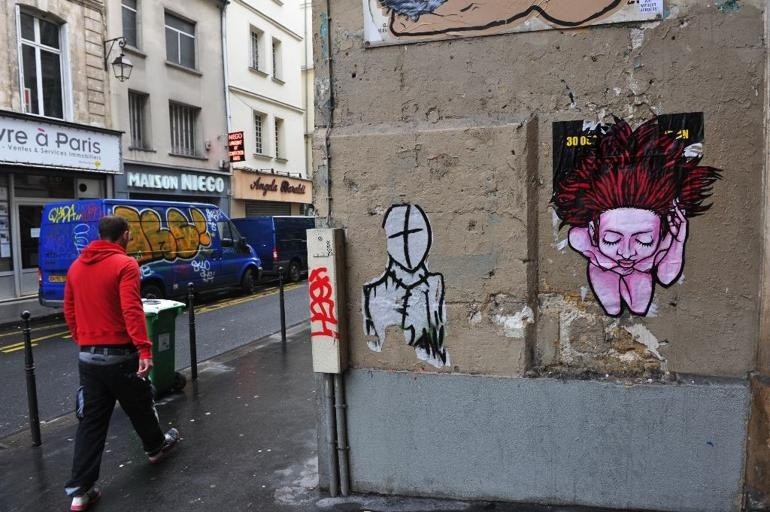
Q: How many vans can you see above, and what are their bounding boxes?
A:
[225,212,314,281]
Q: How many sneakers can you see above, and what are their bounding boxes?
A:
[70,487,103,511]
[148,427,180,464]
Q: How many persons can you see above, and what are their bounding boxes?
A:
[58,215,182,511]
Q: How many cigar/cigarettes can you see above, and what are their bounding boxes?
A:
[136,372,146,380]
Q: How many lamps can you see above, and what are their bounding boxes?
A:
[100,34,134,84]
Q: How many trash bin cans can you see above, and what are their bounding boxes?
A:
[141,298,187,401]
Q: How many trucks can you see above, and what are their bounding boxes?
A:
[30,194,265,314]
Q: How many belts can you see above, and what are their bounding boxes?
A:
[80,345,138,358]
